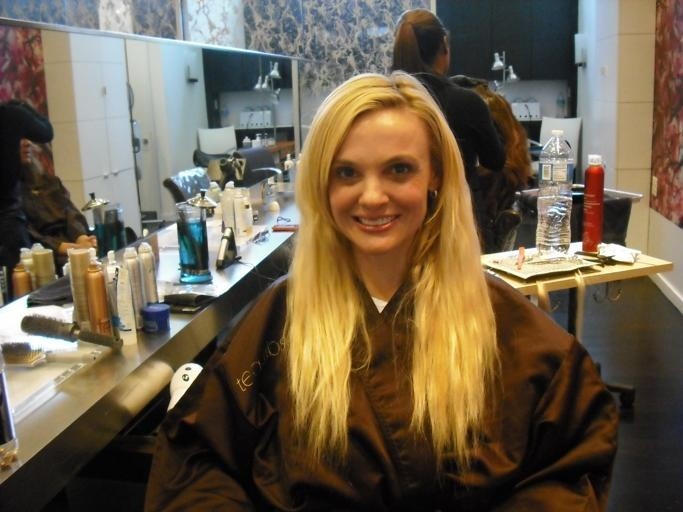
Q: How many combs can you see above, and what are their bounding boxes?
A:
[21,314,123,351]
[1,343,96,368]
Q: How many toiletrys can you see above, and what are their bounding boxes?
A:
[209,182,221,202]
[284,154,294,171]
[242,134,276,149]
[12,243,56,299]
[220,180,254,238]
[69,242,170,336]
[115,268,138,346]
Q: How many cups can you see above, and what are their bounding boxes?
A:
[92,205,126,259]
[175,202,213,284]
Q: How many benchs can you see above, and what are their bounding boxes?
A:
[163,167,211,203]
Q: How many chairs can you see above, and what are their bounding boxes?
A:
[528,117,581,172]
[194,125,237,168]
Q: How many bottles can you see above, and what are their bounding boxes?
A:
[535,130,574,257]
[556,91,566,118]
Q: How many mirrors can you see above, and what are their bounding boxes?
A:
[0,16,301,308]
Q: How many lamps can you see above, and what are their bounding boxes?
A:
[490,51,518,92]
[254,62,282,104]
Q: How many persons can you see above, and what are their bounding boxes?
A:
[391,8,532,252]
[165,71,619,511]
[0,100,97,304]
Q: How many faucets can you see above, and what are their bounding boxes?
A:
[251,166,285,184]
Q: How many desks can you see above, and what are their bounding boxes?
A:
[478,239,672,407]
